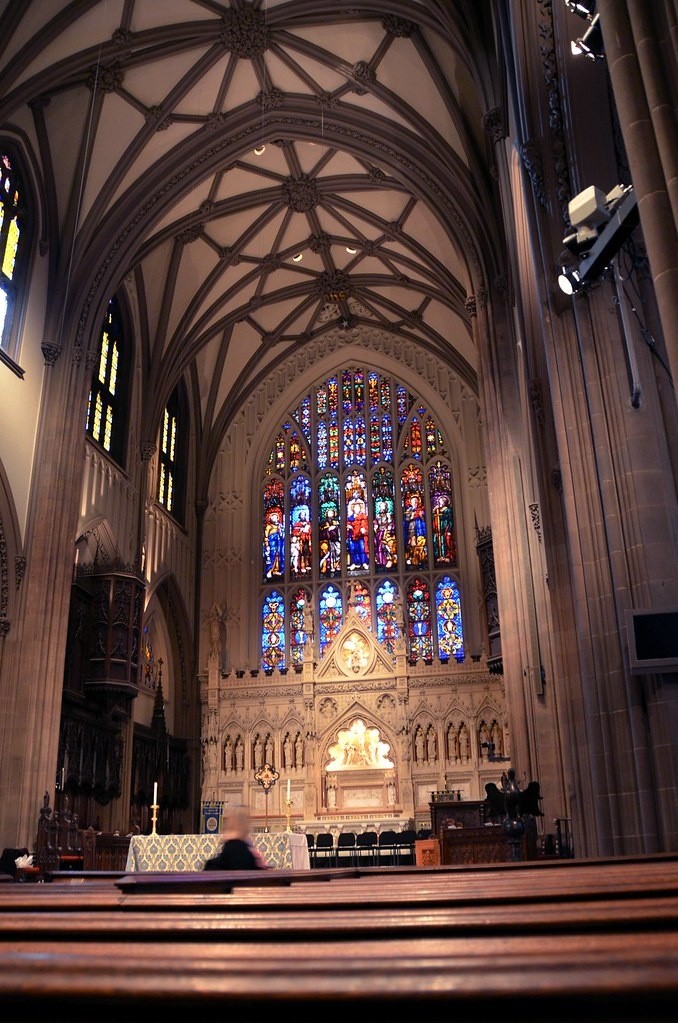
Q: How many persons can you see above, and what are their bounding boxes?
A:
[224,736,303,768]
[485,783,514,821]
[204,807,264,871]
[414,725,501,761]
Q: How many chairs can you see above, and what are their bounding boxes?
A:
[315,833,334,869]
[304,833,316,870]
[336,828,434,869]
[35,791,85,870]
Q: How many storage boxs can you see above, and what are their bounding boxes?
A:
[414,838,440,866]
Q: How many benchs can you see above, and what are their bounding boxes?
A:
[0,851,678,1023]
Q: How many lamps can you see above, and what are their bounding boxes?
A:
[556,183,639,296]
[561,0,602,63]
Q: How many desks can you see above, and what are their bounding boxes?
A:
[124,831,311,871]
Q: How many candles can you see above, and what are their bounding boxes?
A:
[286,778,291,801]
[153,780,158,806]
